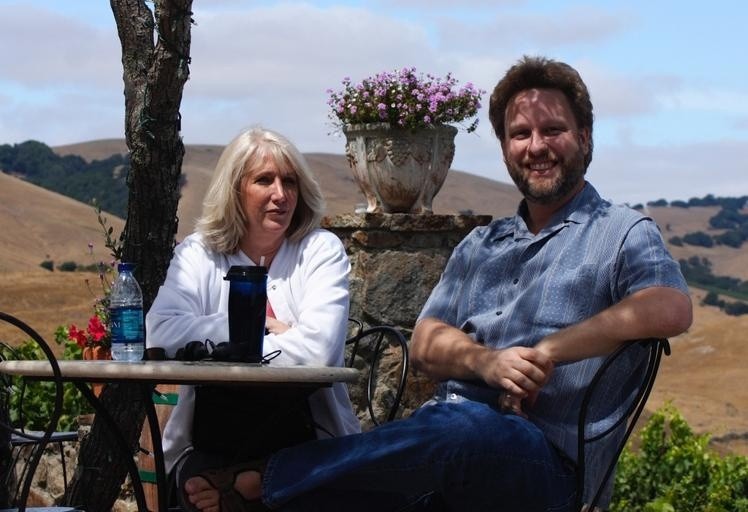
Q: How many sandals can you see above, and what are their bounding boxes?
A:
[179,463,265,512]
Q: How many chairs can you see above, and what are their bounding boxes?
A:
[0,309,78,510]
[575,338,673,512]
[344,325,410,427]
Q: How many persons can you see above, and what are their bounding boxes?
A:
[180,57,695,512]
[145,129,363,489]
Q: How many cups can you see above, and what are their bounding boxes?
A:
[222,264,269,360]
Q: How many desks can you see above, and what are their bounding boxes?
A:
[0,358,360,511]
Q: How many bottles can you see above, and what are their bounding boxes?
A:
[109,262,144,361]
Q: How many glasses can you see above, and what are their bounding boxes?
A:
[143,348,184,361]
[185,339,234,360]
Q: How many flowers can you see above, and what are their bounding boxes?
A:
[324,64,483,136]
[66,244,113,346]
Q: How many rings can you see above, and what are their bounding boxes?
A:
[505,394,512,398]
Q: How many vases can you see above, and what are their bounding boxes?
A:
[343,121,459,215]
[82,346,113,397]
